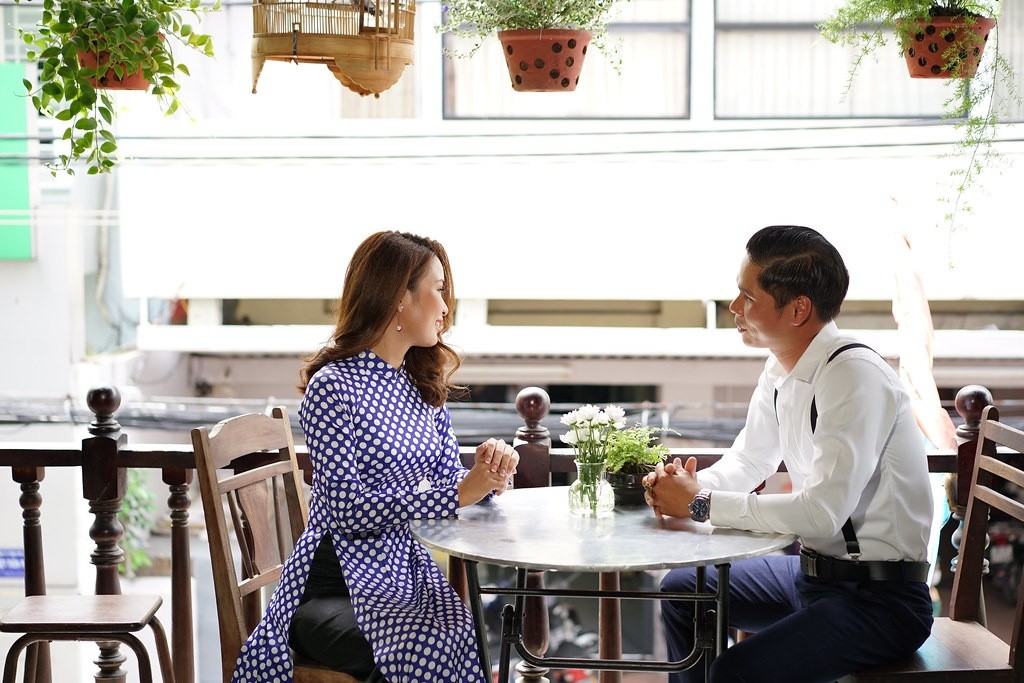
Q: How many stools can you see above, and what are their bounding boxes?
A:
[0,594,176,683]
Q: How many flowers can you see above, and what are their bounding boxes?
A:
[431,0,634,77]
[559,403,627,513]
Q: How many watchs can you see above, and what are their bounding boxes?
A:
[688,488,712,523]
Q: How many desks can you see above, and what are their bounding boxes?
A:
[408,484,800,683]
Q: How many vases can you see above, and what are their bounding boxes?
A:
[495,26,593,92]
[567,460,616,517]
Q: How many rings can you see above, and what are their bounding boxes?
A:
[644,482,652,492]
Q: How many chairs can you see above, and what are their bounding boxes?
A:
[191,405,357,683]
[837,404,1024,683]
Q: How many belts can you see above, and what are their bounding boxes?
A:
[799,551,930,583]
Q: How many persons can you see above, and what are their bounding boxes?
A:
[229,230,521,683]
[641,226,935,683]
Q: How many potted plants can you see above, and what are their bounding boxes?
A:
[814,0,1024,238]
[604,421,682,508]
[7,0,224,179]
